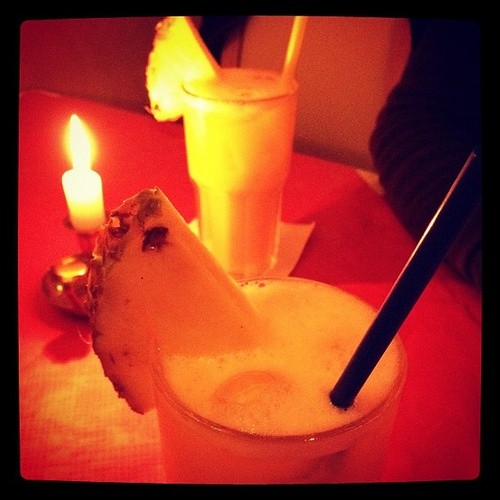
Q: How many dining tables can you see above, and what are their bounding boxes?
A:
[15,88,480,479]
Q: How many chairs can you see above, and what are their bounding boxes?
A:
[276,17,413,172]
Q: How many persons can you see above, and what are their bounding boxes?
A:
[369,15,487,287]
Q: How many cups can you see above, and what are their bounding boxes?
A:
[179,68,299,277]
[147,274,412,490]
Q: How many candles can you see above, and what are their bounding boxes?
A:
[55,107,101,226]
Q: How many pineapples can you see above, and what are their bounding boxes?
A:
[146,16,223,121]
[84,187,261,415]
